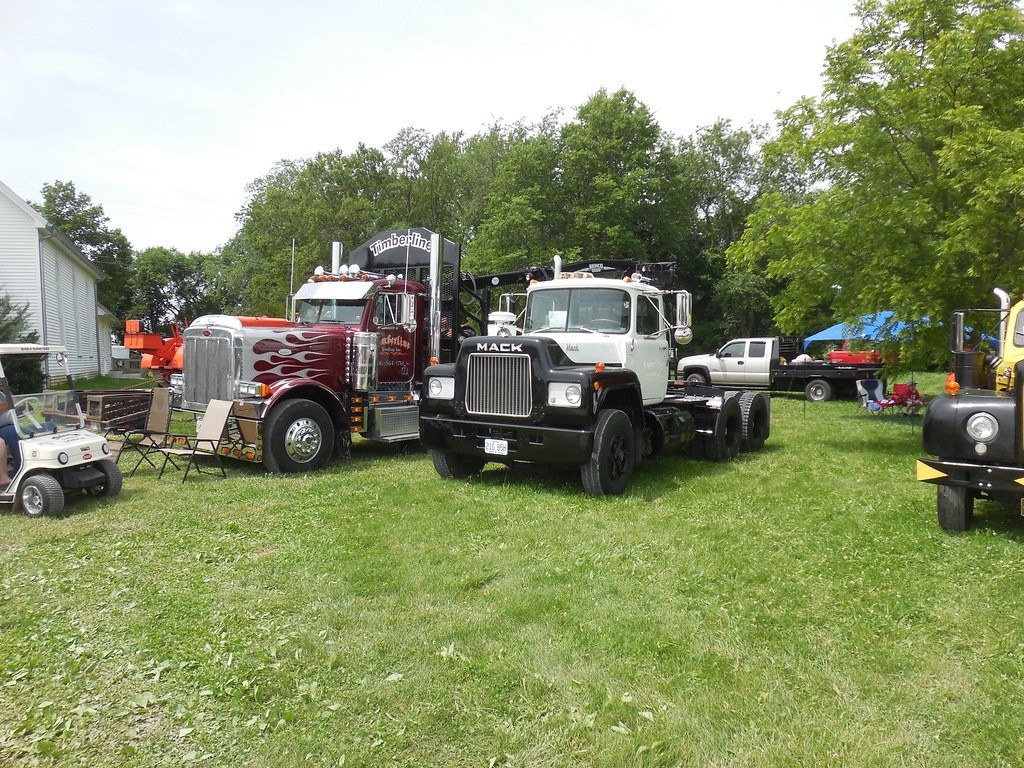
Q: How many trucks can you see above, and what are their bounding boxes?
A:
[916,287,1024,531]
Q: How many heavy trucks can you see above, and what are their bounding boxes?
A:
[168,227,527,474]
[417,254,771,497]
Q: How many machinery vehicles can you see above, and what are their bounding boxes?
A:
[123,317,189,388]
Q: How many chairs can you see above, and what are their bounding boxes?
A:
[104,388,180,477]
[893,383,916,417]
[855,379,896,419]
[156,398,236,484]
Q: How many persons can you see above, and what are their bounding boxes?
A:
[901,380,923,417]
[0,377,57,489]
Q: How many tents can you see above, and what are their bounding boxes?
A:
[803,311,999,420]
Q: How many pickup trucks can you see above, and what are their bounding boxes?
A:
[677,336,888,402]
[828,339,883,363]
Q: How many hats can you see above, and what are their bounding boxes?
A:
[908,381,918,386]
